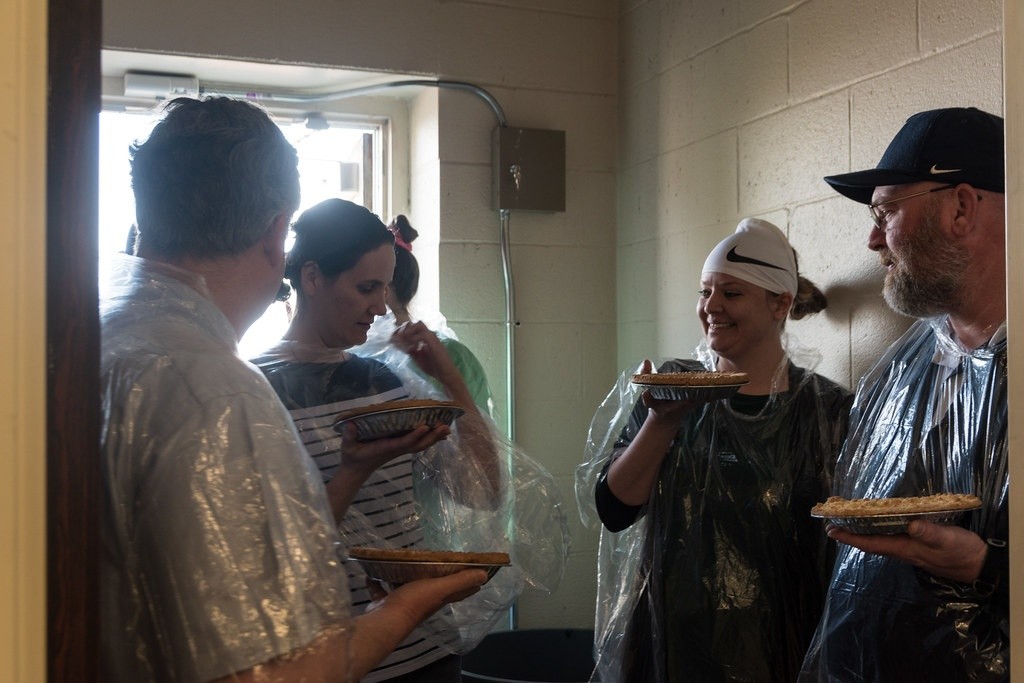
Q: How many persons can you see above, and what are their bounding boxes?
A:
[593,217,857,682]
[817,107,1011,683]
[340,211,488,551]
[245,199,508,683]
[97,94,488,682]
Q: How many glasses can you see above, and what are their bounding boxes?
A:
[869,184,957,229]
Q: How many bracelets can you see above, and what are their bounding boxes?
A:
[954,536,1006,599]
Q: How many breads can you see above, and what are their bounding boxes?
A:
[332,399,465,442]
[346,548,512,586]
[631,370,752,400]
[810,495,982,536]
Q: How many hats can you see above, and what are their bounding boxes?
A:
[825,107,1004,205]
